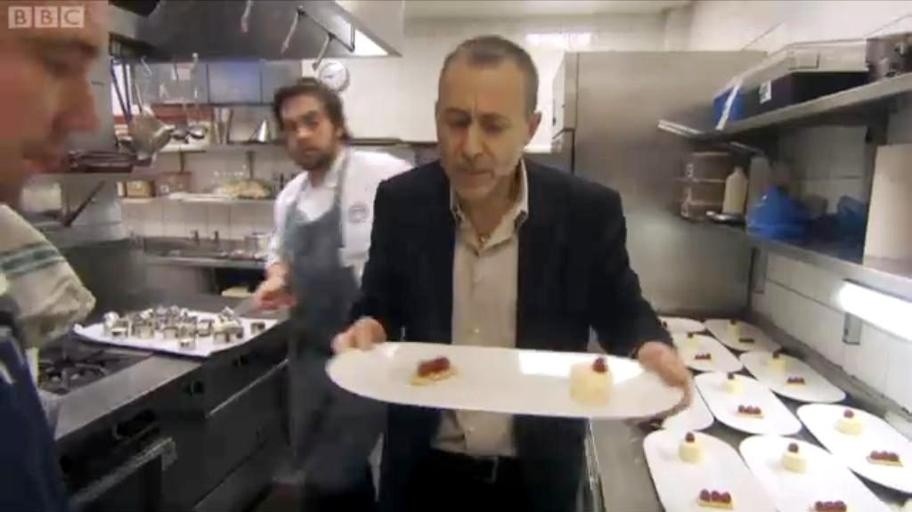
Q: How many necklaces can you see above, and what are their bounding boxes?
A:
[478,235,489,246]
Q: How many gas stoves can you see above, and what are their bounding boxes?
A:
[34,340,153,396]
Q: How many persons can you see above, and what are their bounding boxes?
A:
[0,1,117,511]
[250,72,425,509]
[327,30,696,510]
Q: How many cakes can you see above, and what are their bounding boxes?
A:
[568,357,612,403]
[406,356,458,387]
[101,306,267,348]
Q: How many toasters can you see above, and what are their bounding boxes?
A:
[56,321,287,512]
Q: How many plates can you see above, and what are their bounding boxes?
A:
[673,333,740,372]
[693,372,802,437]
[701,320,781,352]
[658,379,714,429]
[640,430,779,512]
[796,402,912,496]
[323,339,684,423]
[734,435,891,512]
[655,313,704,333]
[739,352,845,404]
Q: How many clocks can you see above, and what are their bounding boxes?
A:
[317,59,347,92]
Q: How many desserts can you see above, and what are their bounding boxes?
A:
[661,318,902,512]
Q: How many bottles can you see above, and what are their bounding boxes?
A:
[720,164,751,217]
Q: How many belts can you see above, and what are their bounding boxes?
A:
[429,447,523,485]
